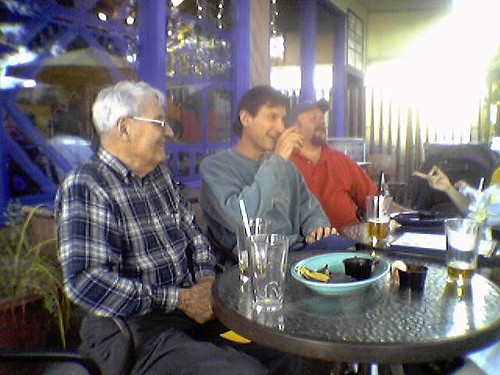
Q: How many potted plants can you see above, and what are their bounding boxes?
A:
[0,197,75,356]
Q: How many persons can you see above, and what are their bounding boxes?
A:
[200,84,339,280]
[287,97,415,235]
[410,163,500,227]
[53,80,270,375]
[37,119,92,183]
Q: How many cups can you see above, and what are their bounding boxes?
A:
[234,218,271,282]
[399,264,428,293]
[444,219,480,286]
[245,234,289,313]
[365,195,393,250]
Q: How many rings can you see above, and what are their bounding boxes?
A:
[205,305,210,311]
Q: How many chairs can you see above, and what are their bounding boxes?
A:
[410,142,500,213]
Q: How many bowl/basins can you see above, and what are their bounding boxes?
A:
[290,252,391,296]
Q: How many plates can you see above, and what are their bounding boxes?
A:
[394,212,456,226]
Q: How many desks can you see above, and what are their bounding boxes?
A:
[342,220,500,266]
[210,249,500,375]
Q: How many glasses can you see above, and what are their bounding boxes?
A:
[129,115,168,127]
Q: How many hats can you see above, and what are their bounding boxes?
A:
[289,97,332,123]
